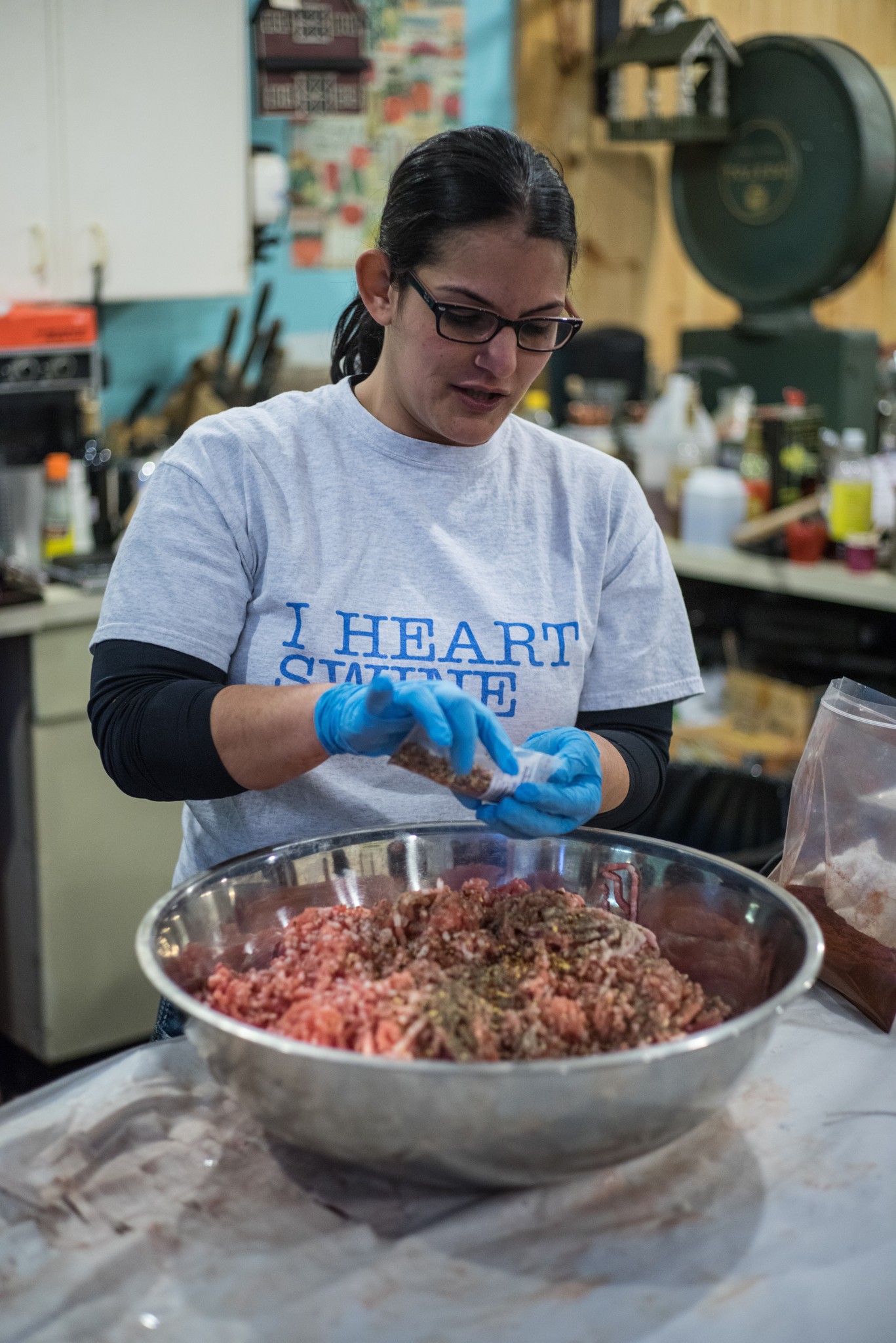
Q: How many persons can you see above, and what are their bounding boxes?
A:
[81,124,706,1053]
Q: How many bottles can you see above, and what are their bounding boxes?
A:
[572,362,896,571]
[41,452,74,568]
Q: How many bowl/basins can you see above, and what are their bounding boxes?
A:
[131,821,825,1190]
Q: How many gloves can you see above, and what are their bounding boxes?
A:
[314,675,519,808]
[477,726,601,840]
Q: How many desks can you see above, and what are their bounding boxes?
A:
[0,964,896,1342]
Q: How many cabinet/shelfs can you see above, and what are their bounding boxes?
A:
[1,581,189,1068]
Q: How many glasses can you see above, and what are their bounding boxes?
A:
[394,269,584,351]
